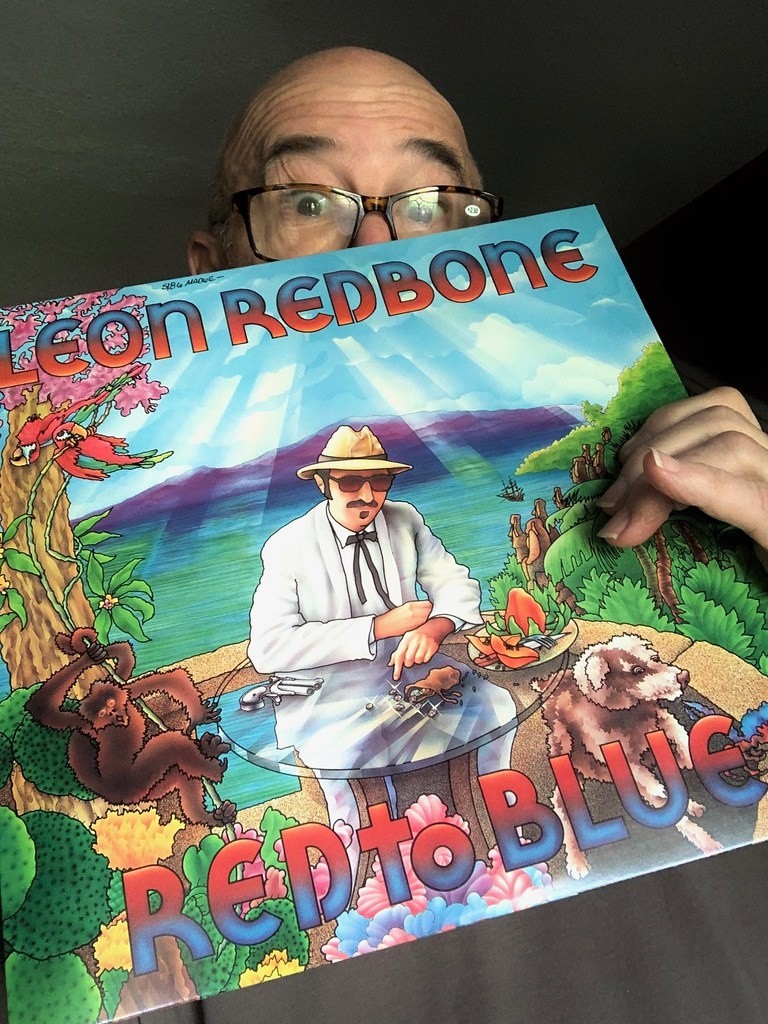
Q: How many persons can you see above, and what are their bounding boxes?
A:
[103,47,768,1024]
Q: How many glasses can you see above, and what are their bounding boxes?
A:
[208,183,503,263]
